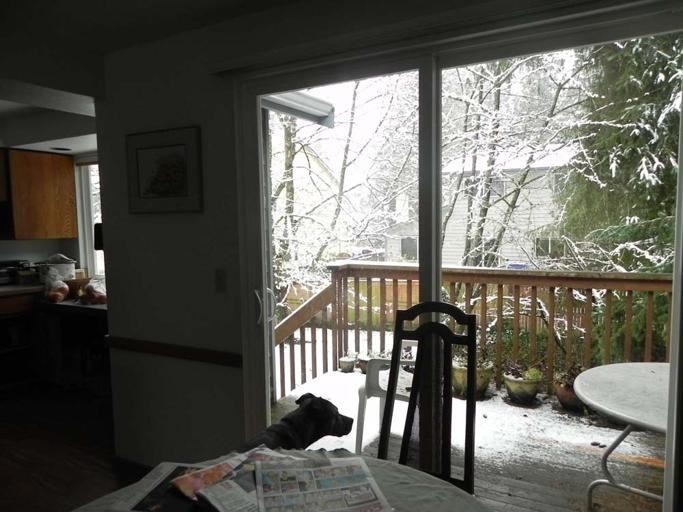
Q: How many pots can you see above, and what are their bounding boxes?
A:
[28,260,77,285]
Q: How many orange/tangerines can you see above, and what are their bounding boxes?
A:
[48,286,67,302]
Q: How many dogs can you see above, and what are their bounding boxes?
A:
[236,392,354,453]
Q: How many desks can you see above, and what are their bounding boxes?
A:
[76,454,492,512]
[572,362,670,512]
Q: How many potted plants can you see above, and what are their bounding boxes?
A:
[451,347,588,414]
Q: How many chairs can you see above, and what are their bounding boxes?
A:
[376,299,477,497]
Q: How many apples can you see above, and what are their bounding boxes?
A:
[89,294,107,304]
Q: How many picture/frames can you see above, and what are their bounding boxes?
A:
[125,125,204,214]
